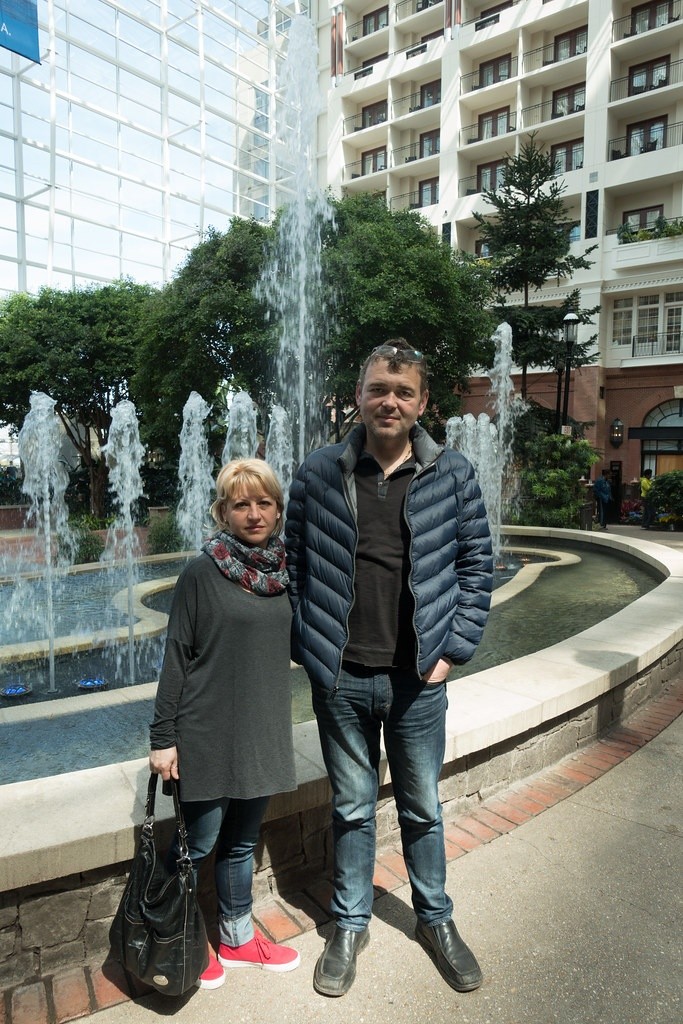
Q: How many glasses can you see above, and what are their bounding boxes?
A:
[368,344,428,364]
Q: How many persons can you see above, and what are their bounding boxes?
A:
[639,468,654,530]
[147,455,305,990]
[285,337,494,997]
[594,467,613,533]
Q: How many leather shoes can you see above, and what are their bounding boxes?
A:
[413,919,485,993]
[313,920,372,998]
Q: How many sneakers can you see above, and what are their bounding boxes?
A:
[193,948,228,991]
[216,931,302,975]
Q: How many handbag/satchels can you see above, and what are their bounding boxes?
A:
[107,770,211,997]
